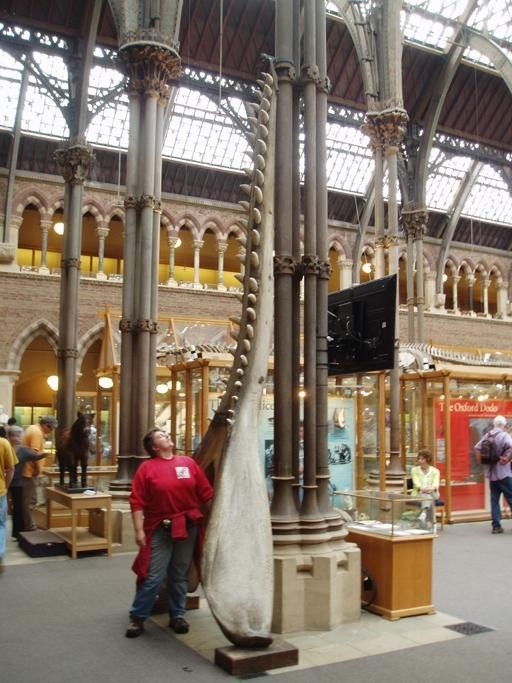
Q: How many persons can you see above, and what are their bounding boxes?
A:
[474,414,512,534]
[124,429,213,637]
[296,422,305,503]
[1,414,58,574]
[410,449,440,530]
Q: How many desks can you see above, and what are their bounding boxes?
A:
[44,485,112,561]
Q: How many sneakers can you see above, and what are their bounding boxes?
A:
[492,525,503,533]
[125,614,146,638]
[169,617,189,634]
[417,512,426,523]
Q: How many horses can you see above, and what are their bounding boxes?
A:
[55,410,96,489]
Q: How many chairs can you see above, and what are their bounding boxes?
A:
[402,477,444,531]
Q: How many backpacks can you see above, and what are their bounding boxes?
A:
[480,430,506,464]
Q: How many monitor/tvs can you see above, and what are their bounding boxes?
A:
[325,271,400,380]
